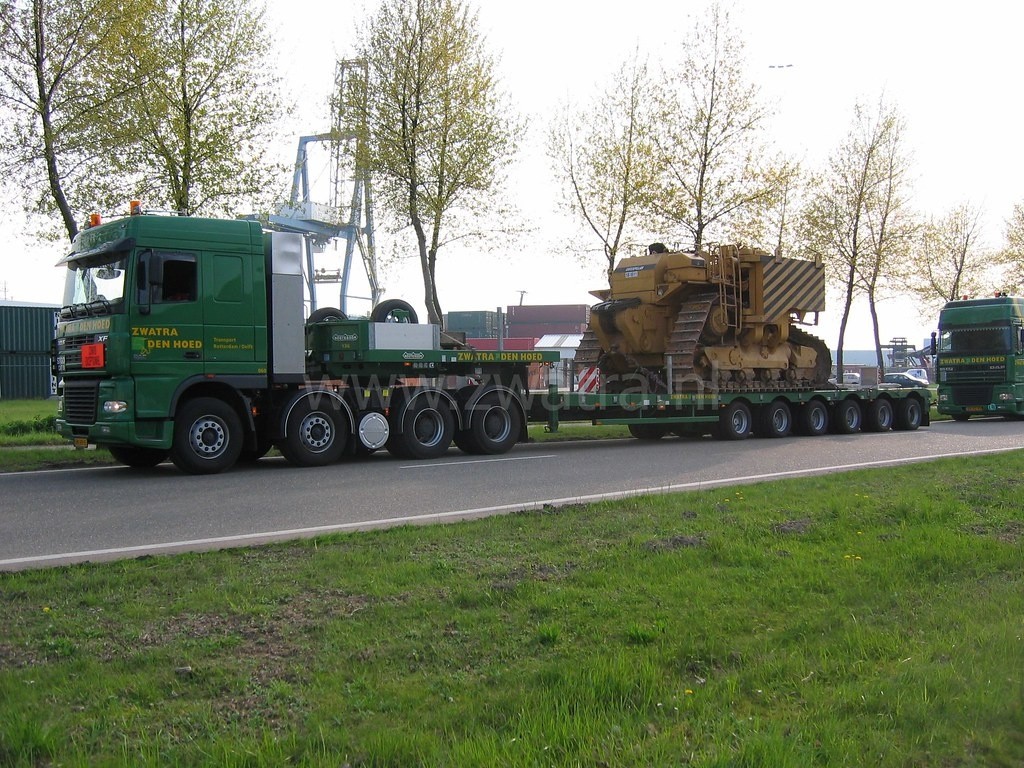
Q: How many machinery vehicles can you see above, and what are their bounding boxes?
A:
[570,240,833,393]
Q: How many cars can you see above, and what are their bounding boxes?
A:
[828,372,862,390]
[883,369,930,388]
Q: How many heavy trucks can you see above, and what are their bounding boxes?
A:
[932,291,1024,422]
[47,200,934,479]
